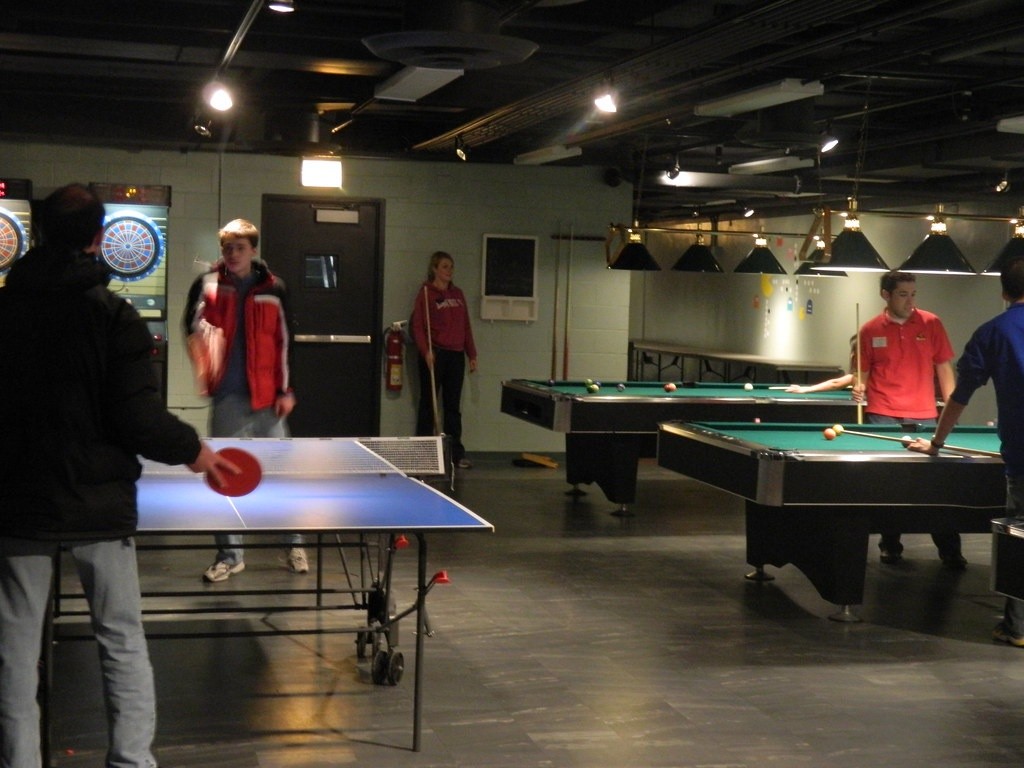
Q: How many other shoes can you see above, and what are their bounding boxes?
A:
[941,553,968,567]
[880,550,901,565]
[457,457,471,468]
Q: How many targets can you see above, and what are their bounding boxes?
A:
[0,207,28,277]
[98,210,164,282]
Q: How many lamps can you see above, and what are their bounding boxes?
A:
[454,136,472,162]
[693,79,824,118]
[512,144,583,166]
[194,114,215,137]
[375,66,465,102]
[809,81,890,273]
[992,178,1010,195]
[980,222,1024,277]
[268,0,294,13]
[897,216,977,276]
[793,239,848,277]
[606,233,663,271]
[742,205,755,217]
[728,154,814,176]
[816,130,840,152]
[670,233,726,274]
[732,236,788,275]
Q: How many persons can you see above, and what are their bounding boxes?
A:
[410,251,477,468]
[906,257,1024,647]
[179,218,309,582]
[785,268,968,568]
[0,183,243,768]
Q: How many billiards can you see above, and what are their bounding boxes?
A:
[832,424,844,436]
[902,436,911,447]
[824,428,835,440]
[547,379,555,386]
[587,384,599,393]
[669,383,676,390]
[595,380,601,386]
[585,379,593,386]
[617,383,625,392]
[744,383,754,391]
[664,385,672,392]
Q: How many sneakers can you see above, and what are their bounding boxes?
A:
[991,622,1024,648]
[286,548,309,574]
[202,557,246,583]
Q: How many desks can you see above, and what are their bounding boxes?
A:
[39,432,495,768]
[629,341,841,383]
[656,420,1007,624]
[500,378,946,516]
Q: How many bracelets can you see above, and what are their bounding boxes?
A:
[931,439,945,448]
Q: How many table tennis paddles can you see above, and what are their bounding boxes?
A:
[206,447,262,498]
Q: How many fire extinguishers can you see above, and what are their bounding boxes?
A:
[383,319,408,392]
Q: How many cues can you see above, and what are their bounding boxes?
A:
[563,225,574,380]
[769,385,853,390]
[424,285,441,436]
[842,430,1002,458]
[551,220,563,380]
[857,302,863,424]
